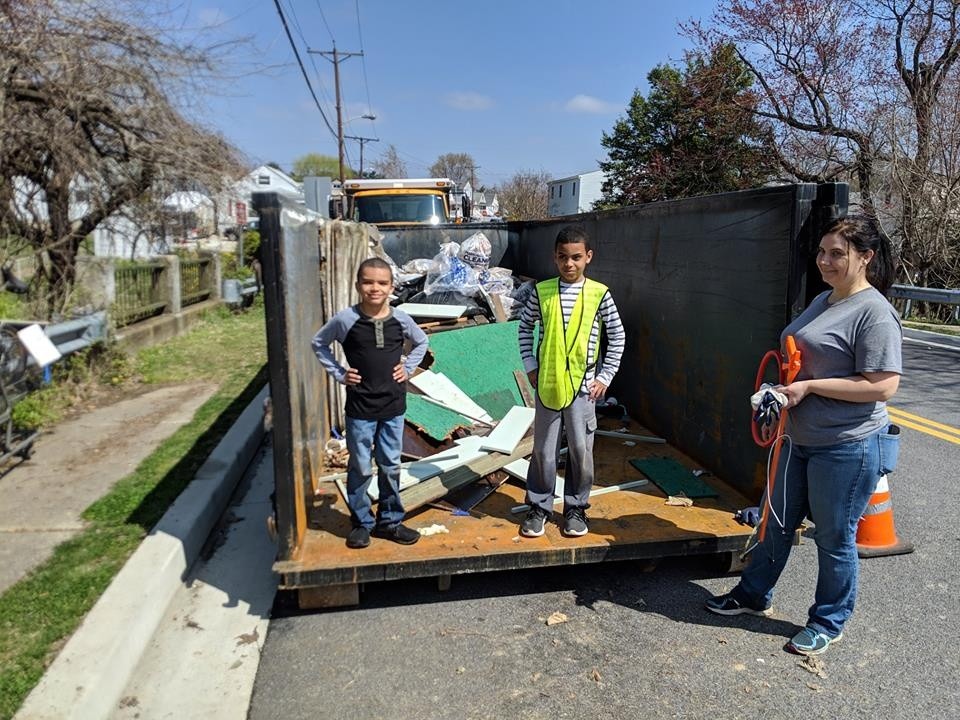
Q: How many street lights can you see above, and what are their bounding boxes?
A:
[338,114,377,195]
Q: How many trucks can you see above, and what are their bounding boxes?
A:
[329,178,471,226]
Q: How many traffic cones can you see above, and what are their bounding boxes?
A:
[856,474,915,559]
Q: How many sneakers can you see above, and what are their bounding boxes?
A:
[348,527,370,548]
[704,592,774,617]
[788,626,843,655]
[521,505,548,536]
[564,508,590,535]
[371,522,420,544]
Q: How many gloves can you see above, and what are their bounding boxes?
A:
[753,382,783,425]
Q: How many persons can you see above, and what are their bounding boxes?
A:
[518,227,626,537]
[311,257,430,548]
[704,216,903,656]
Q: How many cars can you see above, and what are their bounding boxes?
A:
[223,220,259,241]
